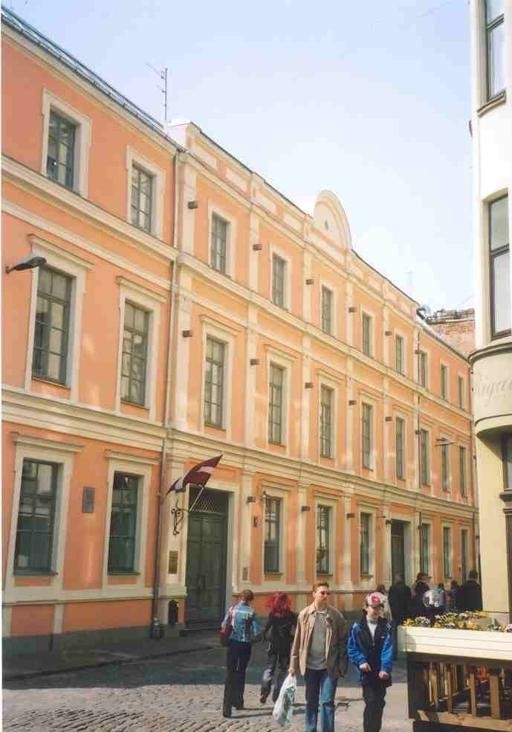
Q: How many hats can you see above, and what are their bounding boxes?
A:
[364,592,388,608]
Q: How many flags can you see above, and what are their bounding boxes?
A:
[164,454,223,494]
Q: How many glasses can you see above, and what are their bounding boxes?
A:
[321,591,330,595]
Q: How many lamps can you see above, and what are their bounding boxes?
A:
[6,255,48,276]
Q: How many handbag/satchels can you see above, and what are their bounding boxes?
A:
[218,604,235,636]
[272,671,297,729]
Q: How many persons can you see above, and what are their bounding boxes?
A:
[363,570,482,634]
[258,592,299,704]
[221,590,262,717]
[346,591,393,732]
[289,581,349,731]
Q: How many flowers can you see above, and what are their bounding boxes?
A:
[403,608,511,636]
